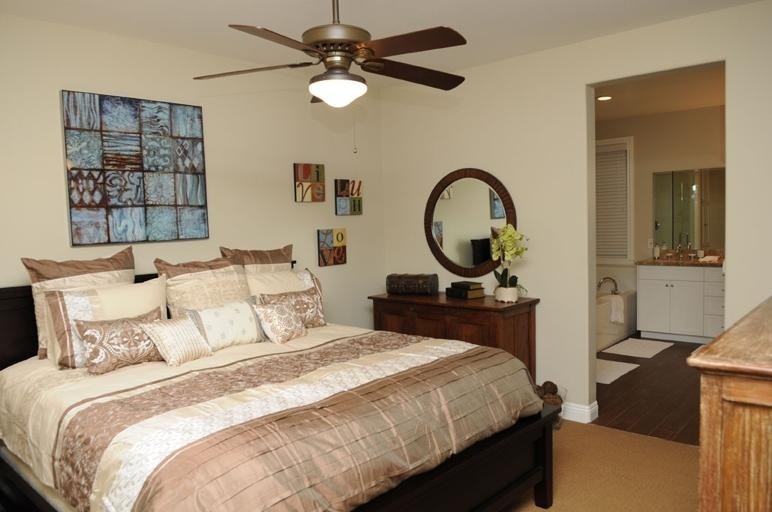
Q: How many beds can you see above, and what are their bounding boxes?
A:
[3,258,565,512]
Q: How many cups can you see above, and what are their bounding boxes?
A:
[696,249,704,259]
[661,248,668,259]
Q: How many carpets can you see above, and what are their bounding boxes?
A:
[602,337,674,358]
[596,359,639,385]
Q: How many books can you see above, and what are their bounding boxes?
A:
[451,281,482,289]
[446,287,485,299]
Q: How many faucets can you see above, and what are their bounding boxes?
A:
[675,245,684,259]
[687,242,691,250]
[596,276,618,295]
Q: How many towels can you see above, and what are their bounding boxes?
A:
[599,294,624,325]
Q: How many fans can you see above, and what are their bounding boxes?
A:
[192,0,467,105]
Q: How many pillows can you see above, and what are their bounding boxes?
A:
[153,257,250,318]
[138,313,214,368]
[44,273,167,367]
[182,300,267,353]
[19,245,137,362]
[243,268,328,345]
[220,244,296,272]
[72,307,163,375]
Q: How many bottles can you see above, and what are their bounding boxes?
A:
[654,242,660,259]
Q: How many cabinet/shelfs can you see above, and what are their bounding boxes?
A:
[366,289,538,383]
[682,295,772,511]
[636,265,702,344]
[701,267,725,343]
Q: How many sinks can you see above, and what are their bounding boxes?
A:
[655,259,684,264]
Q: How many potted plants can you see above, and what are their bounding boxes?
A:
[485,226,524,303]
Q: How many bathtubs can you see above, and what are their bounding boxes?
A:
[596,290,637,354]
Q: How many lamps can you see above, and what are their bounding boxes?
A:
[304,71,370,109]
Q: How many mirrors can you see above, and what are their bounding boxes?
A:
[424,167,518,278]
[650,167,725,254]
